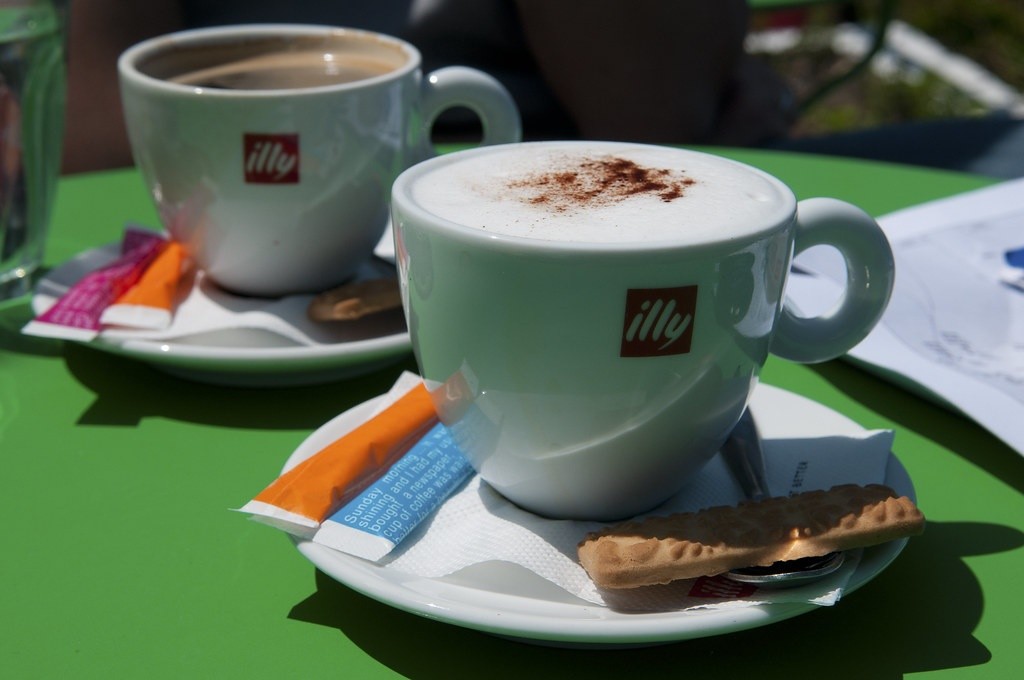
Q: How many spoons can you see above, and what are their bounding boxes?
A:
[722,407,846,587]
[308,277,398,323]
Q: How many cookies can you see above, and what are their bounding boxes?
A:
[307,277,400,321]
[574,482,926,589]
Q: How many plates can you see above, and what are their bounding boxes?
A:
[782,178,1024,458]
[281,391,918,641]
[32,215,412,387]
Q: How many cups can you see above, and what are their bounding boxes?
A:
[119,22,523,294]
[0,0,68,303]
[392,140,896,522]
